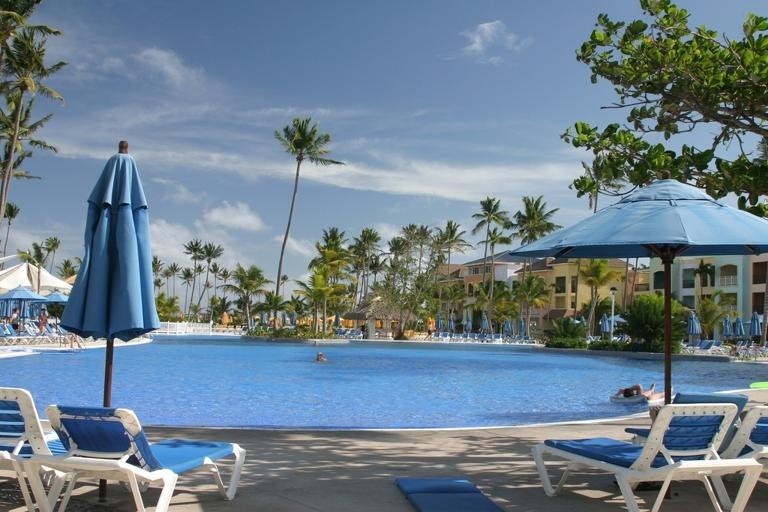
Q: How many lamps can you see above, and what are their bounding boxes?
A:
[0,384,248,508]
[1,309,82,344]
[424,308,644,347]
[675,327,767,363]
[529,388,767,512]
[238,305,377,340]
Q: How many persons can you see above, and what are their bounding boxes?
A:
[37,306,49,336]
[9,306,21,335]
[423,320,433,340]
[615,383,676,400]
[316,352,325,361]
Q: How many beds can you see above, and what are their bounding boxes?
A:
[0,284,48,318]
[568,314,628,331]
[447,313,455,341]
[688,309,702,349]
[464,311,472,340]
[251,308,341,329]
[599,313,610,343]
[481,311,489,339]
[749,310,762,360]
[518,318,524,342]
[30,287,71,333]
[508,171,768,500]
[436,314,442,340]
[722,313,734,345]
[503,319,513,343]
[59,141,161,501]
[735,316,746,344]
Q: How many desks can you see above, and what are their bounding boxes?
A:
[607,286,618,342]
